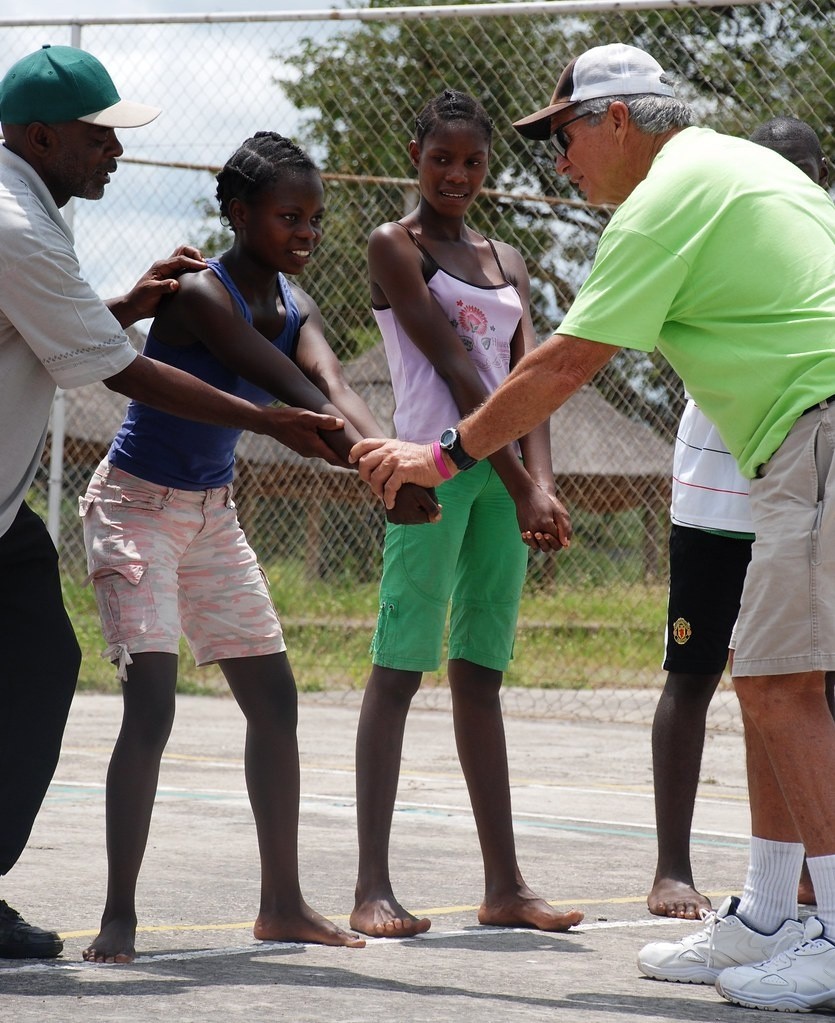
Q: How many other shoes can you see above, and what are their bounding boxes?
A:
[0,899,64,959]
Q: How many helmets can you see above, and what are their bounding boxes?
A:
[748,117,828,186]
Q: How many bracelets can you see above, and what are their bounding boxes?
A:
[433,441,451,480]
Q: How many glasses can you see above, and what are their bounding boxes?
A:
[540,110,592,163]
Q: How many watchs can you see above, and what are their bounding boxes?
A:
[440,427,478,470]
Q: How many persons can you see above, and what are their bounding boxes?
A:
[0,42,352,961]
[648,116,833,919]
[78,130,364,962]
[348,88,586,935]
[347,41,834,1010]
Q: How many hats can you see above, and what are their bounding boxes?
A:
[0,44,163,128]
[511,42,676,140]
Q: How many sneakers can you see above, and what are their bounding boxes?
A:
[715,915,835,1013]
[634,894,805,986]
